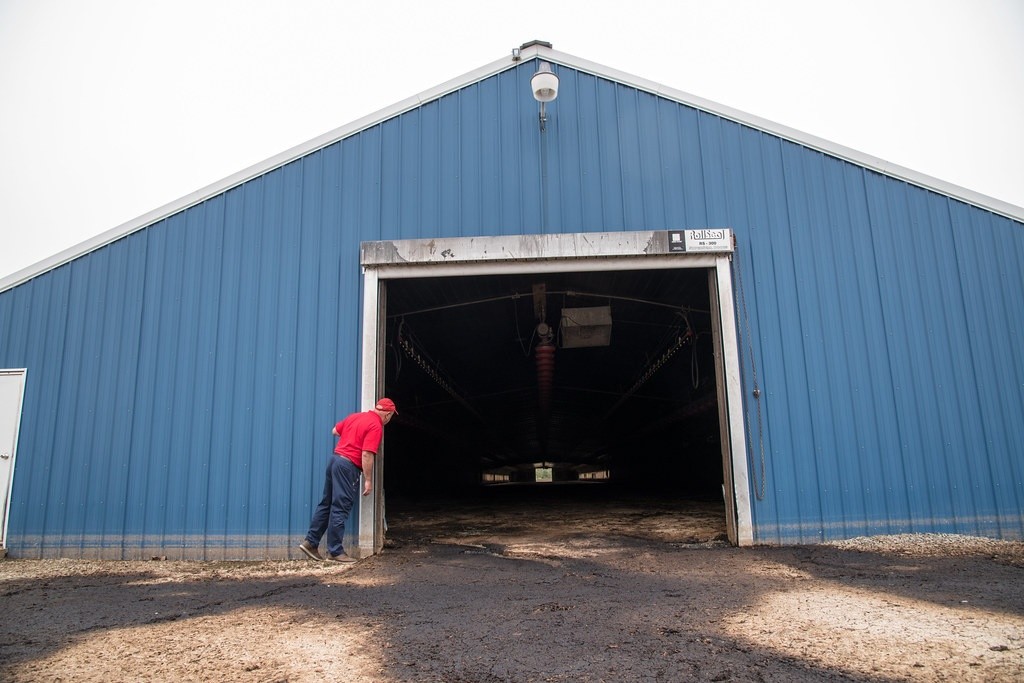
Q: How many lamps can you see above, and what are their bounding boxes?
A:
[529,61,561,132]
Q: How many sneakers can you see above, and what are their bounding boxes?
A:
[326,552,357,565]
[299,540,324,561]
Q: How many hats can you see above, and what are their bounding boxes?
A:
[376,398,400,416]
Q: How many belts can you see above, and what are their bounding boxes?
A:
[335,453,352,462]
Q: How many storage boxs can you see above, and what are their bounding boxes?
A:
[560,306,614,348]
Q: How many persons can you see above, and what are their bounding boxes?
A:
[299,397,399,565]
